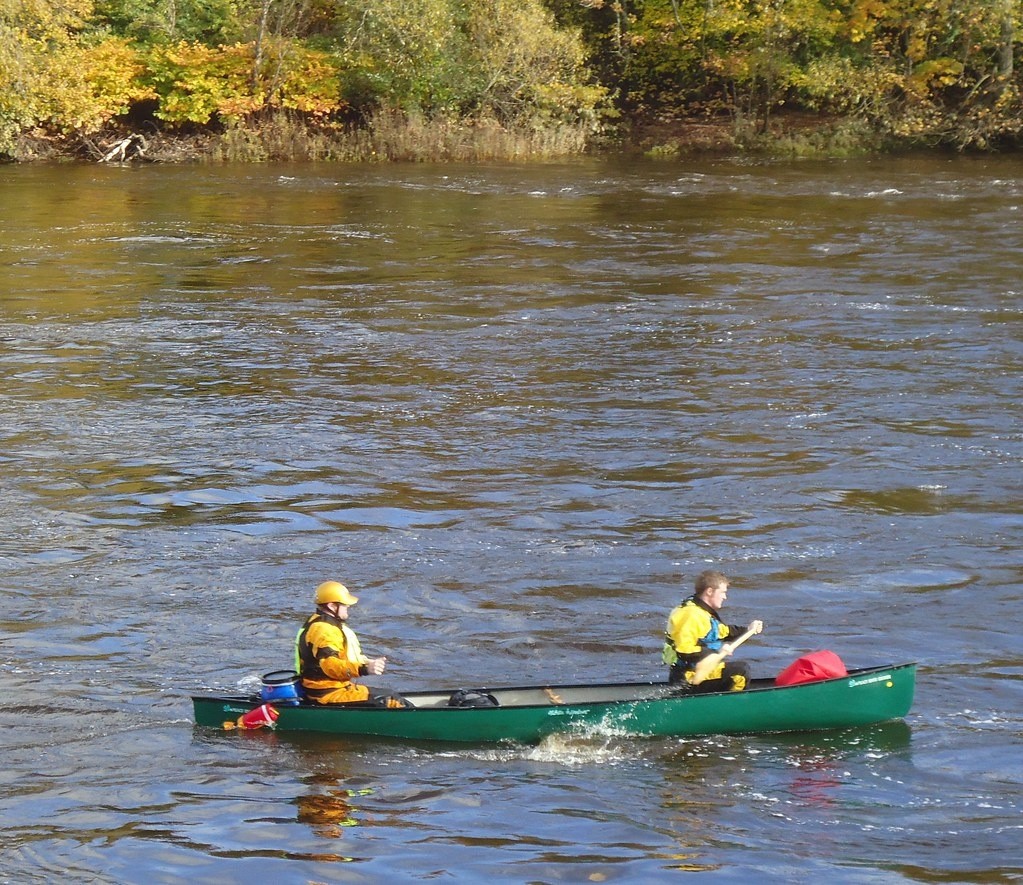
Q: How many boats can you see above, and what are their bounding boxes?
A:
[190,661,918,743]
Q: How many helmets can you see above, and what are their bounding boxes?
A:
[315,581,359,606]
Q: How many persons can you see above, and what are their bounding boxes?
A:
[661,569,765,692]
[295,580,414,707]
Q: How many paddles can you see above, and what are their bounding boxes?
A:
[691,624,759,686]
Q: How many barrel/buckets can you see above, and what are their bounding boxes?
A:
[259,670,304,706]
